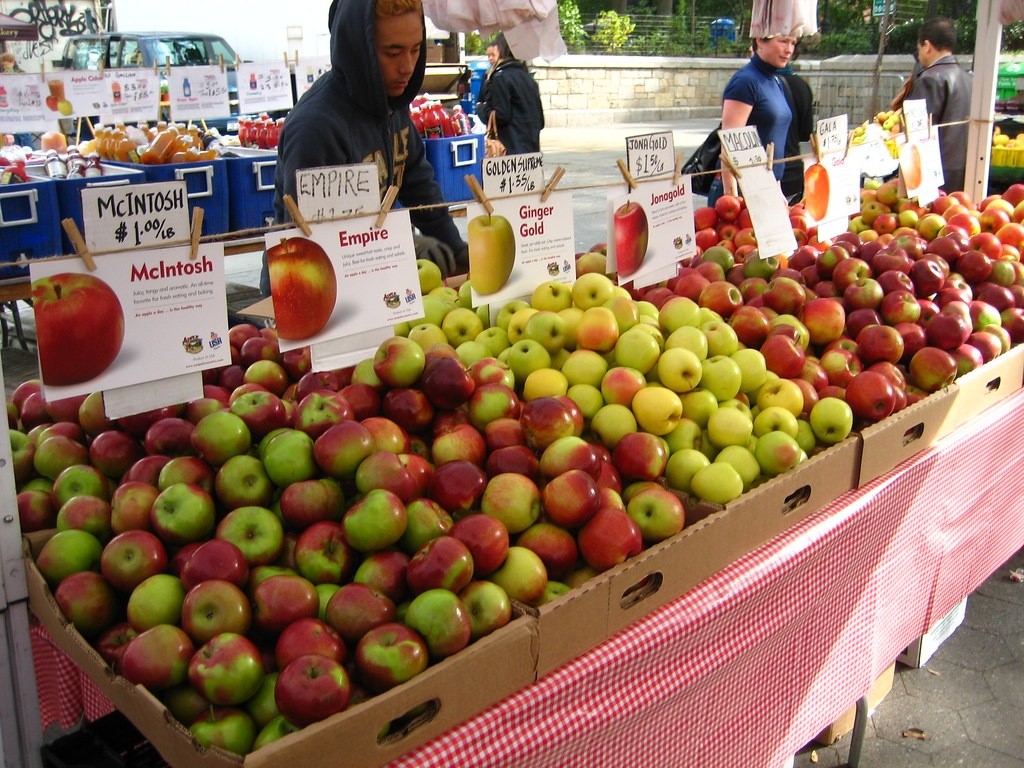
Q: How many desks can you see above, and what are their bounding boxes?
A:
[31,391,1024,768]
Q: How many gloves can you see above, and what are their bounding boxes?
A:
[413,234,454,281]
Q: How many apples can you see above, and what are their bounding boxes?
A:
[804,164,829,221]
[613,199,649,277]
[385,244,854,509]
[991,131,1024,151]
[468,212,516,295]
[898,142,922,188]
[848,107,902,158]
[689,179,1024,434]
[267,236,337,340]
[32,273,126,387]
[7,322,686,758]
[45,95,72,115]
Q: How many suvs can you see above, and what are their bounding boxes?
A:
[55,30,292,150]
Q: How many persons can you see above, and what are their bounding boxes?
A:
[749,47,813,205]
[707,36,800,208]
[0,53,35,151]
[476,34,545,154]
[259,0,469,291]
[905,18,972,195]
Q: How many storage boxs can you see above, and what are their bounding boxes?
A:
[511,437,861,677]
[225,155,279,230]
[424,133,485,202]
[0,168,62,275]
[851,343,1024,486]
[99,158,229,237]
[26,161,147,255]
[20,529,536,766]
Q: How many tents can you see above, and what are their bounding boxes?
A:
[0,13,38,41]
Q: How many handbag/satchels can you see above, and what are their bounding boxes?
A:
[681,123,723,196]
[890,77,913,111]
[483,111,506,158]
